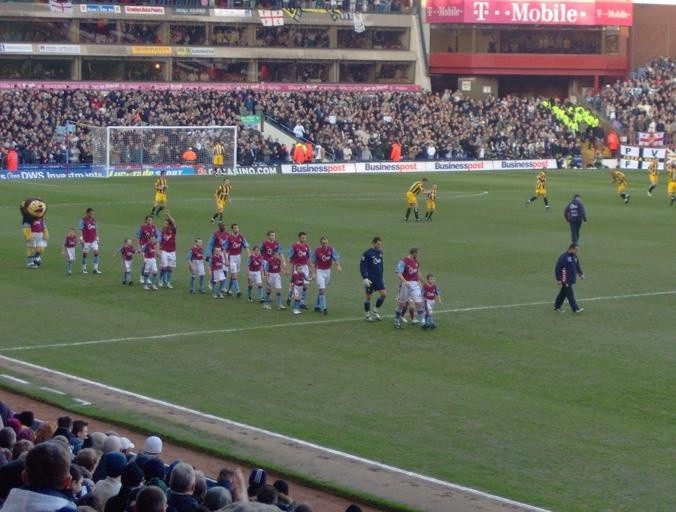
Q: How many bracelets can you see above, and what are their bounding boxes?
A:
[96,236,99,240]
[79,236,83,240]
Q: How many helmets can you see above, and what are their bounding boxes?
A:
[143,436,163,454]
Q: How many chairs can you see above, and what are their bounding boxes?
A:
[0,410,222,512]
[0,16,675,173]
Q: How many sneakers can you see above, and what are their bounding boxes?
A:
[190,280,242,299]
[248,296,328,315]
[553,306,564,313]
[574,308,584,314]
[80,269,102,274]
[122,273,174,291]
[365,310,436,330]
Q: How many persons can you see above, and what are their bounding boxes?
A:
[149,169,169,217]
[553,244,586,314]
[401,177,430,223]
[118,215,177,291]
[79,207,106,274]
[0,2,675,178]
[345,504,362,512]
[666,153,676,206]
[562,194,589,246]
[187,221,342,314]
[423,184,440,222]
[208,178,233,224]
[359,237,441,331]
[524,167,553,214]
[647,159,659,197]
[609,170,630,204]
[1,401,314,512]
[61,227,85,276]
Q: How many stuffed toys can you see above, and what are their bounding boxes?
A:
[20,197,50,270]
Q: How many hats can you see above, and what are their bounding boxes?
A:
[249,468,266,485]
[119,436,136,450]
